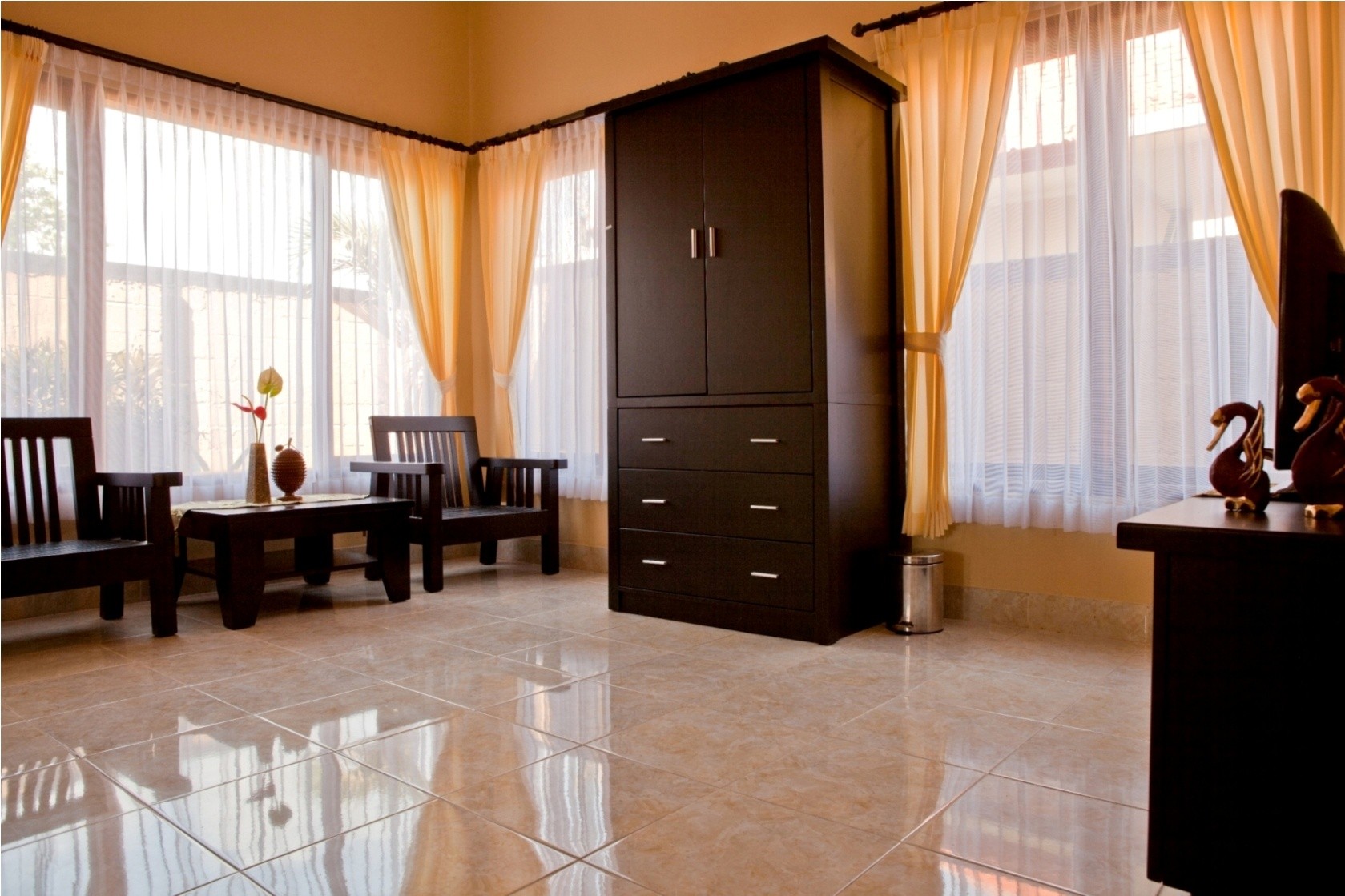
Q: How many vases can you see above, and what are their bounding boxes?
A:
[246,442,271,504]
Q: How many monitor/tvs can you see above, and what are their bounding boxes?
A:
[1277,188,1343,468]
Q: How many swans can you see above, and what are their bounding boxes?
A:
[1205,399,1273,511]
[1290,375,1345,518]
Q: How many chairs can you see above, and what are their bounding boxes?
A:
[0,414,181,638]
[348,413,572,592]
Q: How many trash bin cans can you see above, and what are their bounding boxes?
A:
[891,551,947,634]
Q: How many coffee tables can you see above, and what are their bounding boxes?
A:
[160,492,415,629]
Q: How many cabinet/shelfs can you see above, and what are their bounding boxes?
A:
[1115,495,1345,896]
[601,36,924,636]
